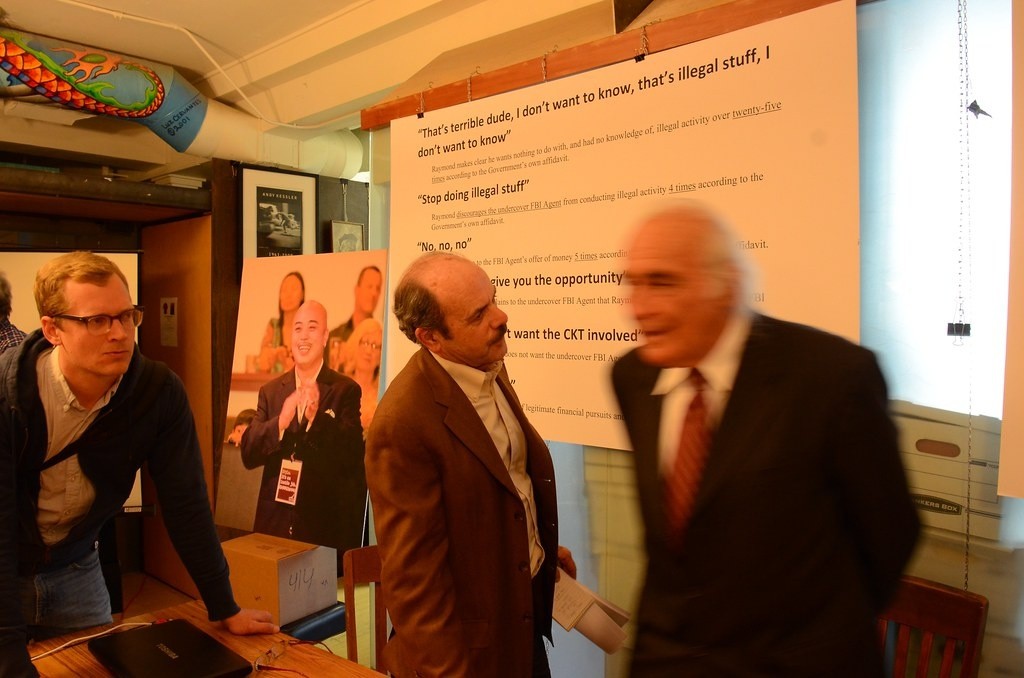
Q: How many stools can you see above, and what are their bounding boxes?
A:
[280,600,347,644]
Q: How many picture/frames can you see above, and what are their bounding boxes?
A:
[330,220,365,253]
[237,164,318,285]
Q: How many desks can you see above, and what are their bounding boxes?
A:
[27,598,392,678]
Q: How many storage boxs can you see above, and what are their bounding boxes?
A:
[221,532,338,629]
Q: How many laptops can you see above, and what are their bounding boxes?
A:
[88,619,253,678]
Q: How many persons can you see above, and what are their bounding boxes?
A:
[0,272,30,353]
[239,261,383,576]
[611,204,927,678]
[0,251,280,678]
[364,254,577,678]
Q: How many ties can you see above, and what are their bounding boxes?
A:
[667,366,710,549]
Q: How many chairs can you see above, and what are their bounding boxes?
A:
[342,545,387,675]
[875,574,990,678]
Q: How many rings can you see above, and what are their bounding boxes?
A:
[309,400,315,404]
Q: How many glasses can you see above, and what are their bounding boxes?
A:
[50,304,145,335]
[360,341,381,352]
